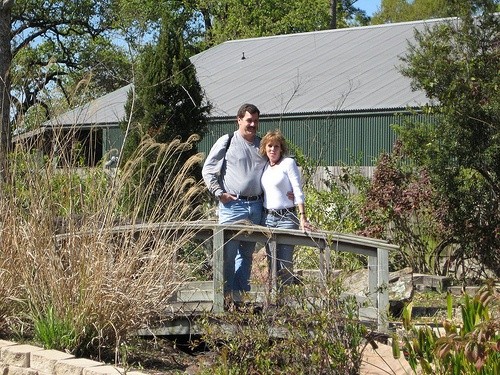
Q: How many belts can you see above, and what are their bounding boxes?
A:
[262,206,296,216]
[231,193,263,201]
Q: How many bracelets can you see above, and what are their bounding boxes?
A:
[298,212,304,215]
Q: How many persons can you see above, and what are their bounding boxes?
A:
[258,130,314,293]
[202,104,295,303]
